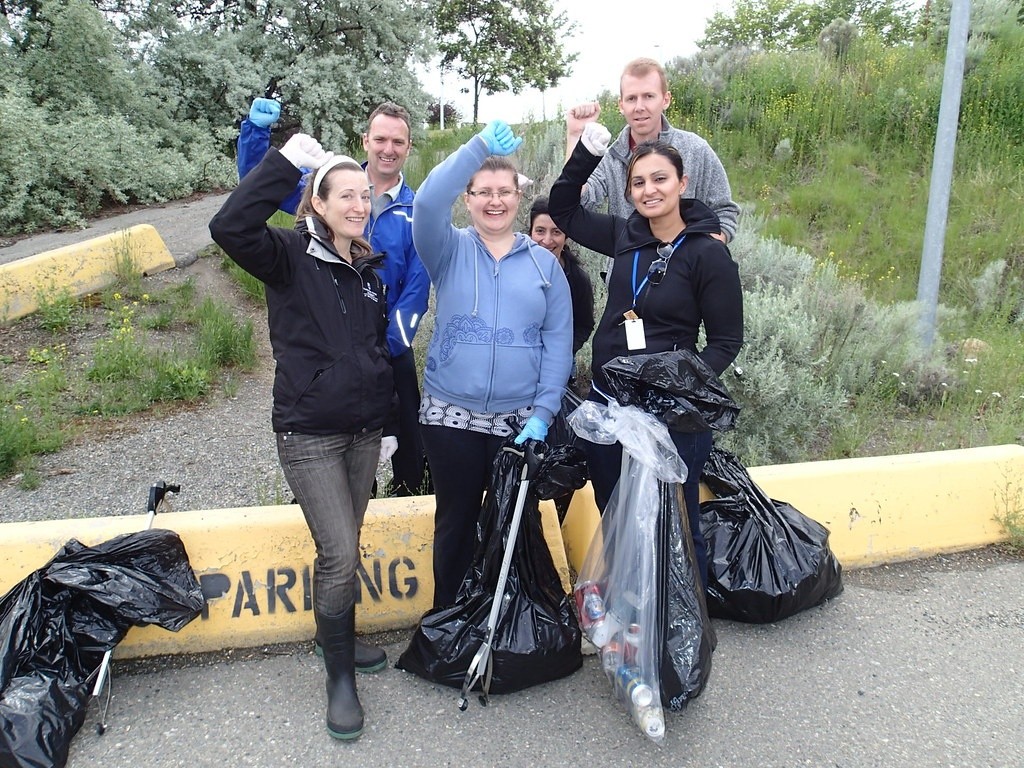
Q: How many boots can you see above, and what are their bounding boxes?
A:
[312,559,386,672]
[313,596,365,740]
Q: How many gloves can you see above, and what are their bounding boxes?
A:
[248,97,281,128]
[514,417,548,444]
[580,123,611,158]
[477,118,522,156]
[278,134,334,170]
[518,172,533,195]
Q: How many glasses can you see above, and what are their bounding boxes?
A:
[467,187,519,200]
[647,241,674,287]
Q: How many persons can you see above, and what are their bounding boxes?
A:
[208,133,393,741]
[526,193,595,394]
[237,97,431,504]
[411,117,573,609]
[564,57,742,297]
[549,121,744,656]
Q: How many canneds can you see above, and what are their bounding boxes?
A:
[574,579,665,739]
